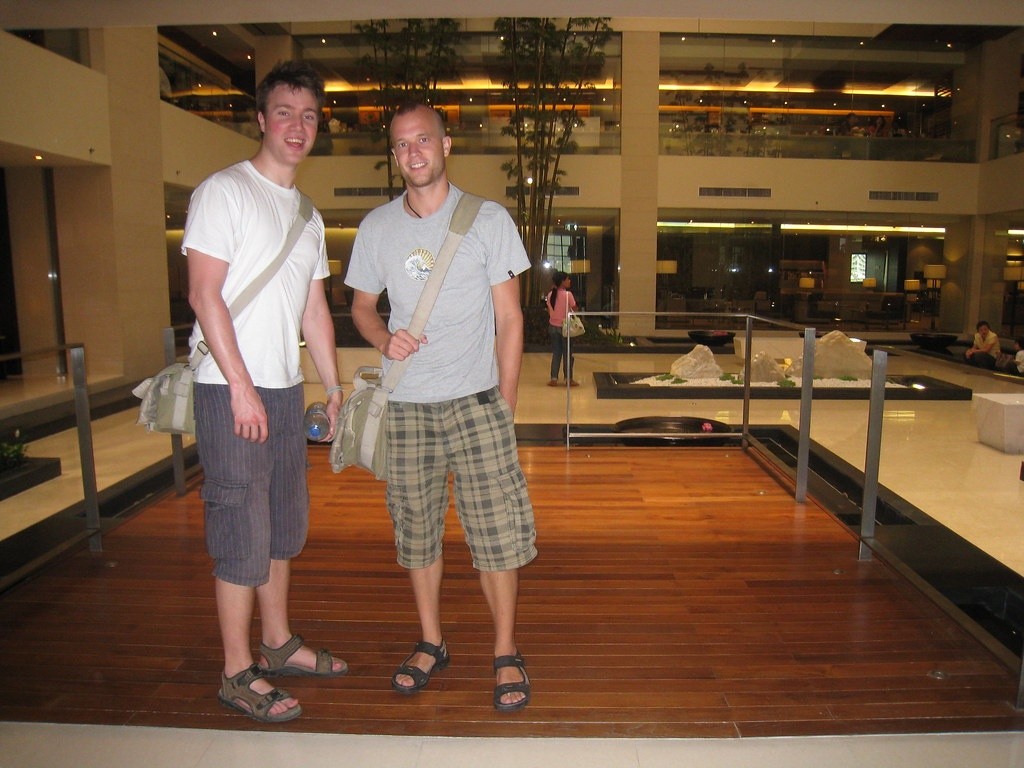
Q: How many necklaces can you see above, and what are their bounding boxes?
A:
[406,193,422,219]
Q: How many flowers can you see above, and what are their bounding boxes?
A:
[712,330,729,336]
[702,423,713,431]
[0,430,31,471]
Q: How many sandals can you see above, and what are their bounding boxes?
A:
[391,638,450,694]
[218,663,303,722]
[259,634,348,677]
[494,650,530,711]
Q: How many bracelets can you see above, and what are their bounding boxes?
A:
[326,386,342,394]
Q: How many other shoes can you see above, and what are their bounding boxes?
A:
[548,381,557,386]
[566,380,579,386]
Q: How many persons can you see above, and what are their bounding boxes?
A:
[181,61,349,722]
[966,321,1001,369]
[838,113,891,138]
[1010,337,1024,377]
[546,272,579,385]
[345,104,537,710]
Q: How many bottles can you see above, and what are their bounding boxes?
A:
[303,401,331,442]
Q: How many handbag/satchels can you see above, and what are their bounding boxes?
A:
[327,366,393,482]
[562,291,585,338]
[132,362,196,434]
[994,351,1015,373]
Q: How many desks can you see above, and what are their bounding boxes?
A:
[779,287,917,323]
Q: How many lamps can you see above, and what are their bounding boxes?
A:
[863,278,877,289]
[924,265,946,330]
[657,260,677,306]
[568,260,590,306]
[799,278,814,291]
[904,280,920,324]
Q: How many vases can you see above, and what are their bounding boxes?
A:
[909,334,958,351]
[687,330,736,347]
[615,416,732,447]
[0,457,61,500]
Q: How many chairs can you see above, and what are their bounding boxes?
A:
[865,296,908,331]
[807,293,841,329]
